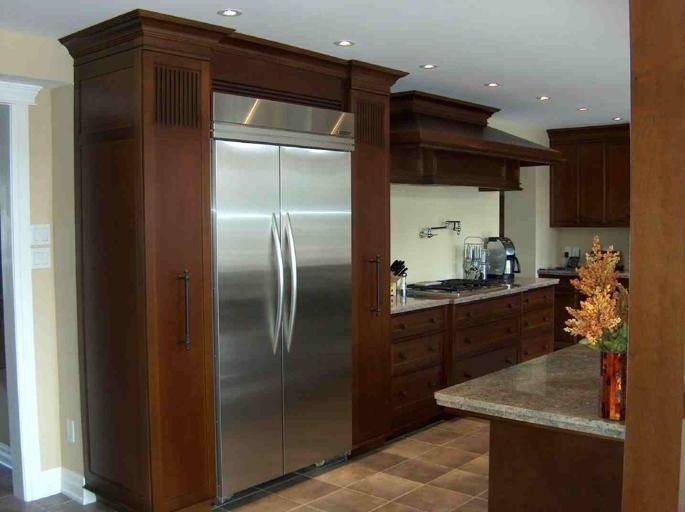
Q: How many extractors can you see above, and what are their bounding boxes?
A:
[389,151,525,193]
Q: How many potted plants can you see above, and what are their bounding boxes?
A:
[561,238,630,422]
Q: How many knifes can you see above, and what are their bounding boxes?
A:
[391,260,408,277]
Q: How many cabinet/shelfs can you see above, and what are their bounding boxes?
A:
[548,138,628,229]
[388,286,556,435]
[72,46,391,512]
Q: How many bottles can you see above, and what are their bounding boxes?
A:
[396,273,408,304]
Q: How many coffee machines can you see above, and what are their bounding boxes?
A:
[483,236,515,289]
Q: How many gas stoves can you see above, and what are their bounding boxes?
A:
[407,279,508,300]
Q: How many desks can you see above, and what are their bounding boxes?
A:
[434,345,627,511]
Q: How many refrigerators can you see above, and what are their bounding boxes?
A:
[209,120,352,503]
